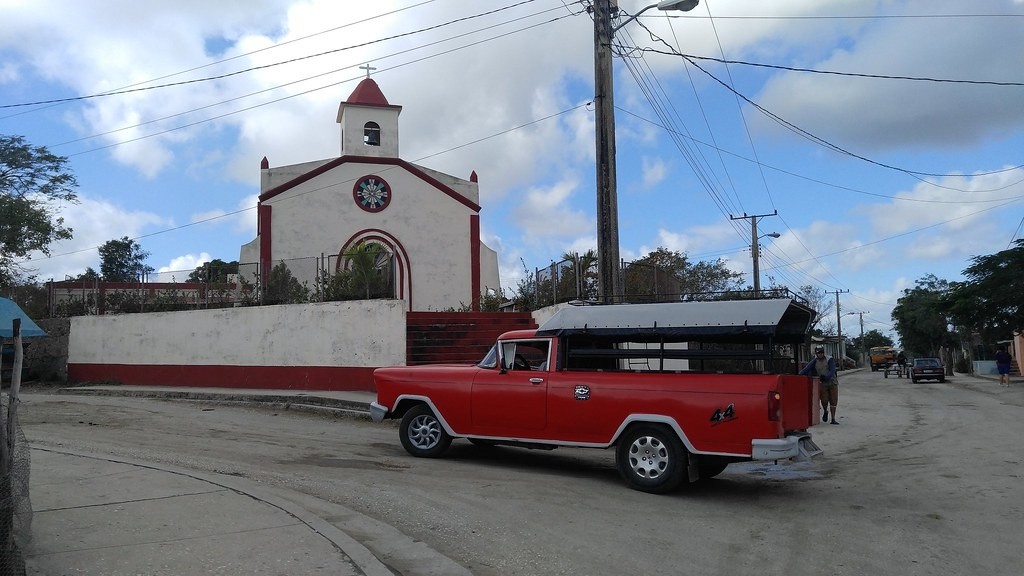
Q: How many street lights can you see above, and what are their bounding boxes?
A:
[837,312,855,371]
[751,232,780,298]
[594,0,700,305]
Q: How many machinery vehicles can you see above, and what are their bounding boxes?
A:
[869,346,896,372]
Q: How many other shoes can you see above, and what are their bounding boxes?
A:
[822,411,828,422]
[830,420,839,424]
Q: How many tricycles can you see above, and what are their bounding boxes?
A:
[884,361,911,379]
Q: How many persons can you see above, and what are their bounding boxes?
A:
[798,345,839,424]
[897,351,907,373]
[992,346,1012,388]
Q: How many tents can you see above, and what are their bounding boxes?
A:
[0,296,49,337]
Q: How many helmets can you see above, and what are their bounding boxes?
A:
[815,345,824,354]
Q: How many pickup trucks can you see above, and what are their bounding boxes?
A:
[369,329,825,493]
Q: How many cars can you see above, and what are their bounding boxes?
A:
[910,358,945,384]
